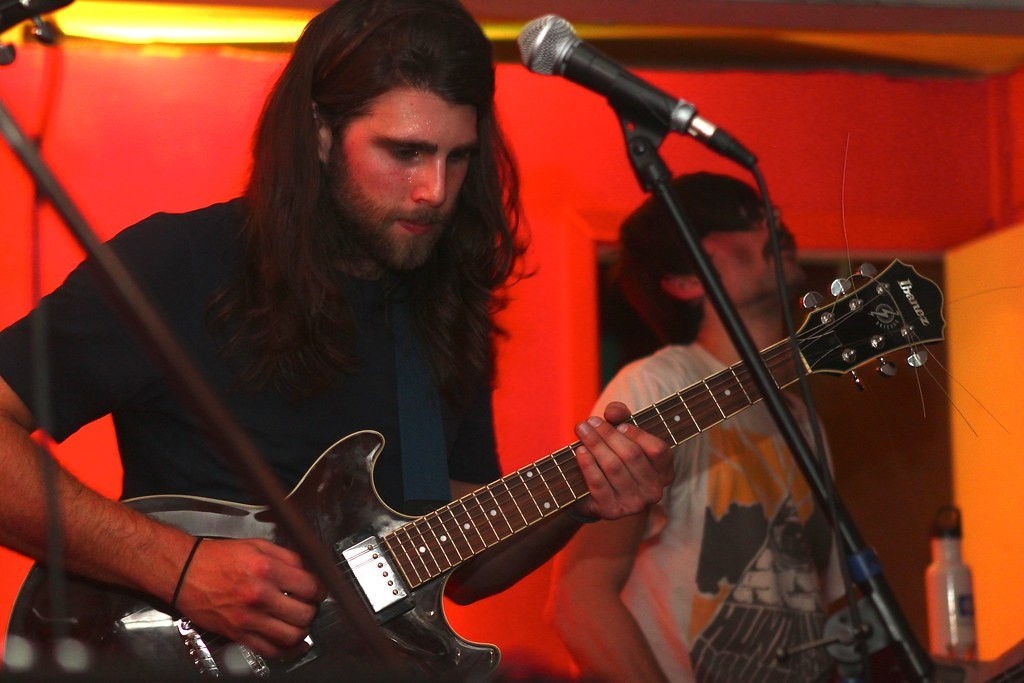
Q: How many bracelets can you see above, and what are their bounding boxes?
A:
[170,537,203,607]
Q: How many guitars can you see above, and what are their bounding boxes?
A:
[2,256,948,682]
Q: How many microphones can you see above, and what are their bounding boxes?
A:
[516,14,758,168]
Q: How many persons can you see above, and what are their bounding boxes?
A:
[1,0,673,683]
[544,173,1023,682]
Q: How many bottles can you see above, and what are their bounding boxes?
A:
[923,506,977,665]
